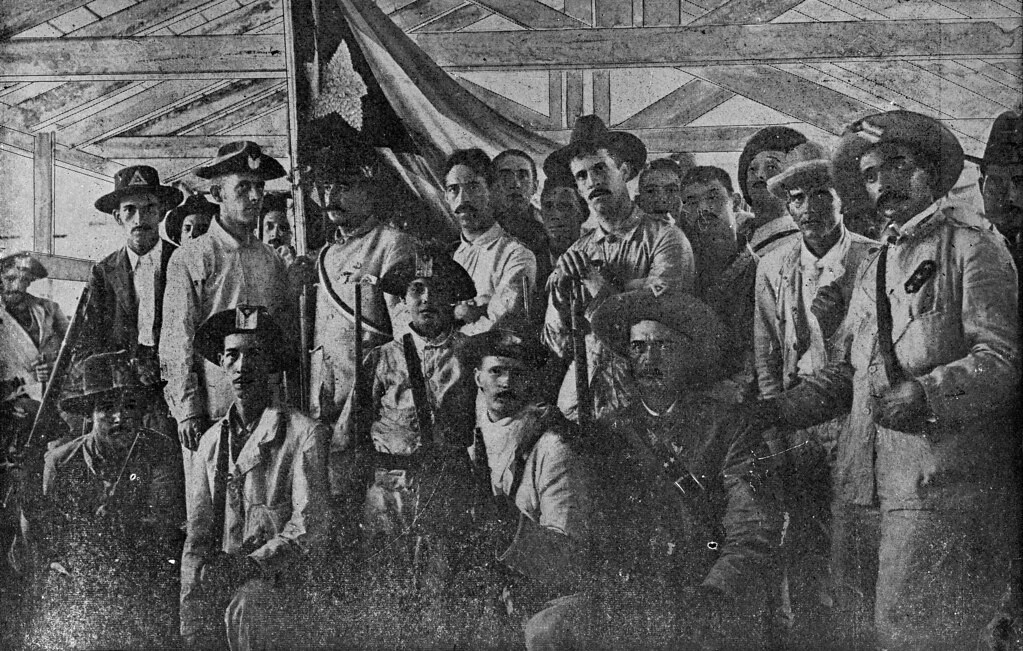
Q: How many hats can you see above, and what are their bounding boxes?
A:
[964,111,1023,164]
[0,245,49,279]
[60,350,169,415]
[192,140,286,181]
[193,307,299,383]
[590,290,724,370]
[543,114,647,188]
[766,143,837,201]
[165,194,220,246]
[462,313,550,370]
[378,244,477,302]
[829,109,965,209]
[739,126,807,206]
[95,166,184,213]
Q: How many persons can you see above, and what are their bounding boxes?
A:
[160,141,302,651]
[0,108,1022,651]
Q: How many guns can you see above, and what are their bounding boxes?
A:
[0,270,609,651]
